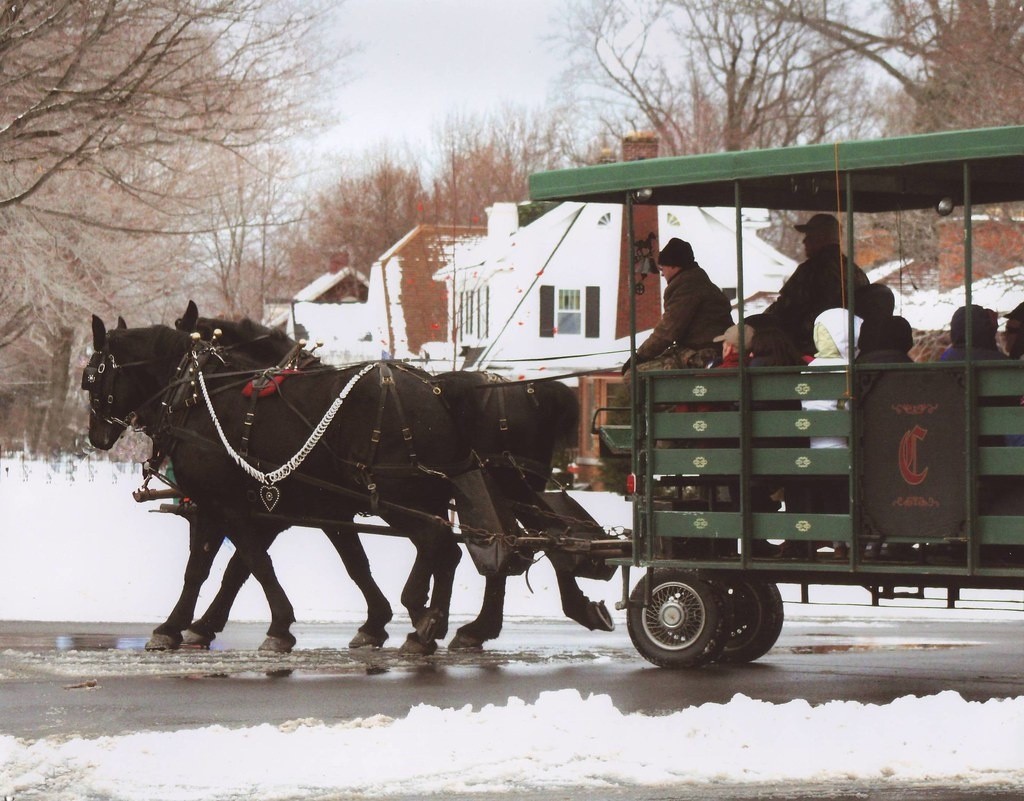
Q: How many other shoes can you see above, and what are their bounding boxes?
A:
[751,539,965,560]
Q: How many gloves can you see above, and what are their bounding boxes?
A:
[621,353,643,376]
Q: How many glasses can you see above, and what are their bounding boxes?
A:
[1007,327,1024,334]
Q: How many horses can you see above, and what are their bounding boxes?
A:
[175,299,616,650]
[80,316,469,654]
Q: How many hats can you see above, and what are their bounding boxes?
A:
[1003,301,1024,321]
[795,214,838,241]
[949,305,995,347]
[712,324,754,351]
[658,238,695,267]
[874,317,913,353]
[857,283,894,322]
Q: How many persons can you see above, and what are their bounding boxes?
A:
[676,213,1024,562]
[619,237,734,452]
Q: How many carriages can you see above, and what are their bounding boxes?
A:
[80,126,1024,669]
